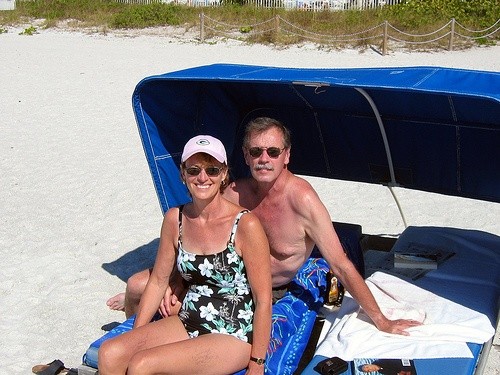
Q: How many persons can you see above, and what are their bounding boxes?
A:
[106,116,424,337]
[98,135,272,374]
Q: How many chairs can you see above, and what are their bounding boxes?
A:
[79,219,500,375]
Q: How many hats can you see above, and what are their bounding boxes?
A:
[181,135,227,166]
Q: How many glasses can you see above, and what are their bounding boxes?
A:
[182,162,225,175]
[243,145,287,157]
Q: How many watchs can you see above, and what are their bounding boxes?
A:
[250,355,266,365]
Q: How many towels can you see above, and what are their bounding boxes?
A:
[313,271,495,362]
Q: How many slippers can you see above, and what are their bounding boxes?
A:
[32,359,78,375]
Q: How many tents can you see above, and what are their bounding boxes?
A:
[84,62,500,375]
